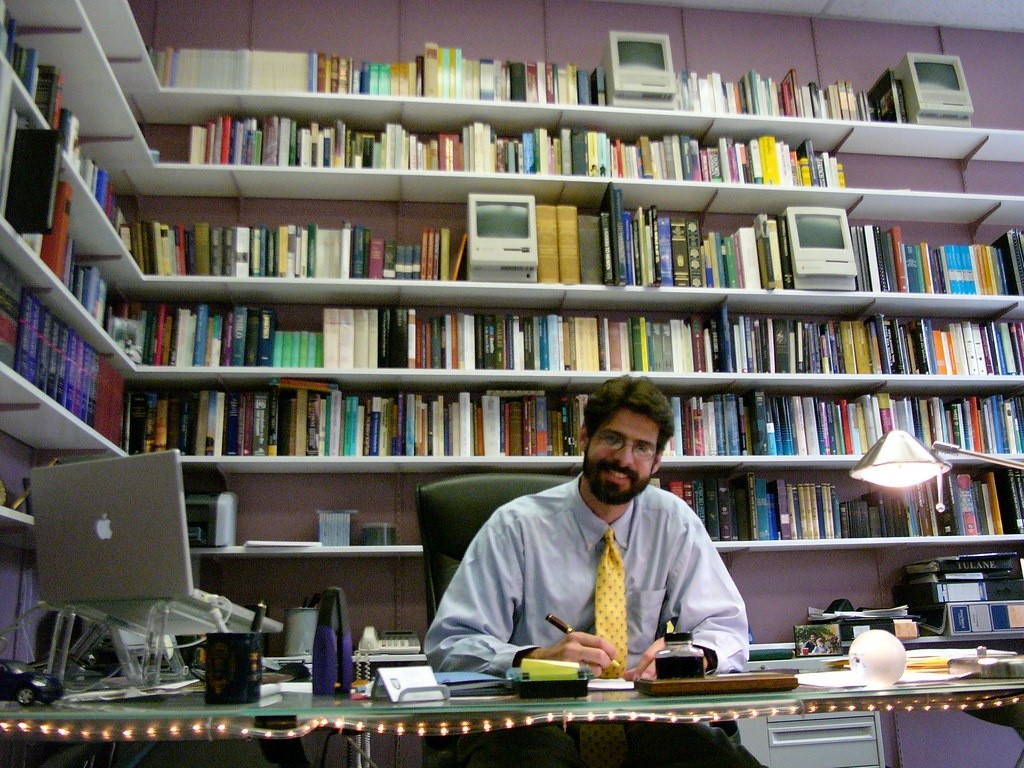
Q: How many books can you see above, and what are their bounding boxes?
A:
[148,40,608,107]
[748,648,795,660]
[853,223,1023,297]
[0,8,160,460]
[188,116,845,188]
[154,219,467,279]
[532,184,792,289]
[806,551,1024,640]
[670,68,908,125]
[149,300,1023,377]
[318,512,360,548]
[143,384,1023,461]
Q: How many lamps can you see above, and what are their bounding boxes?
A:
[846,428,1023,488]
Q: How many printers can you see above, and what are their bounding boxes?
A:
[180,466,240,547]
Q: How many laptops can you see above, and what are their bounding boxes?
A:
[30,449,283,634]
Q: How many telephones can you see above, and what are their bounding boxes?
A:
[358,626,422,655]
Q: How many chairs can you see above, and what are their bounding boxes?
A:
[414,472,590,642]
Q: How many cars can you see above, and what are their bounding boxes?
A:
[0,658,65,708]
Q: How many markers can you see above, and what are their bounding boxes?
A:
[310,593,320,607]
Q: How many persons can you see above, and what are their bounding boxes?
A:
[802,631,829,655]
[423,374,768,768]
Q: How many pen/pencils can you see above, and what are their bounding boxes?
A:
[251,602,268,632]
[545,613,620,668]
[210,608,228,633]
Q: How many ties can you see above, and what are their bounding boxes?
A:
[579,527,630,768]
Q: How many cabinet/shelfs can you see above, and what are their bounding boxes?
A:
[0,1,1024,768]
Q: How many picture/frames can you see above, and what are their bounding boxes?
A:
[792,623,844,657]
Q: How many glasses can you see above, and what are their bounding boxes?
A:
[598,428,658,461]
